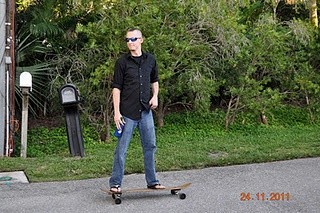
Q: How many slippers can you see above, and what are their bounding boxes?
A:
[146,183,165,189]
[110,184,122,193]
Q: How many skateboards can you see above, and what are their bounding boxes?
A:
[100,182,192,205]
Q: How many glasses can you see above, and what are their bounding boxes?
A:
[126,37,140,42]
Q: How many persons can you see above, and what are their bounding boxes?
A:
[109,28,165,193]
